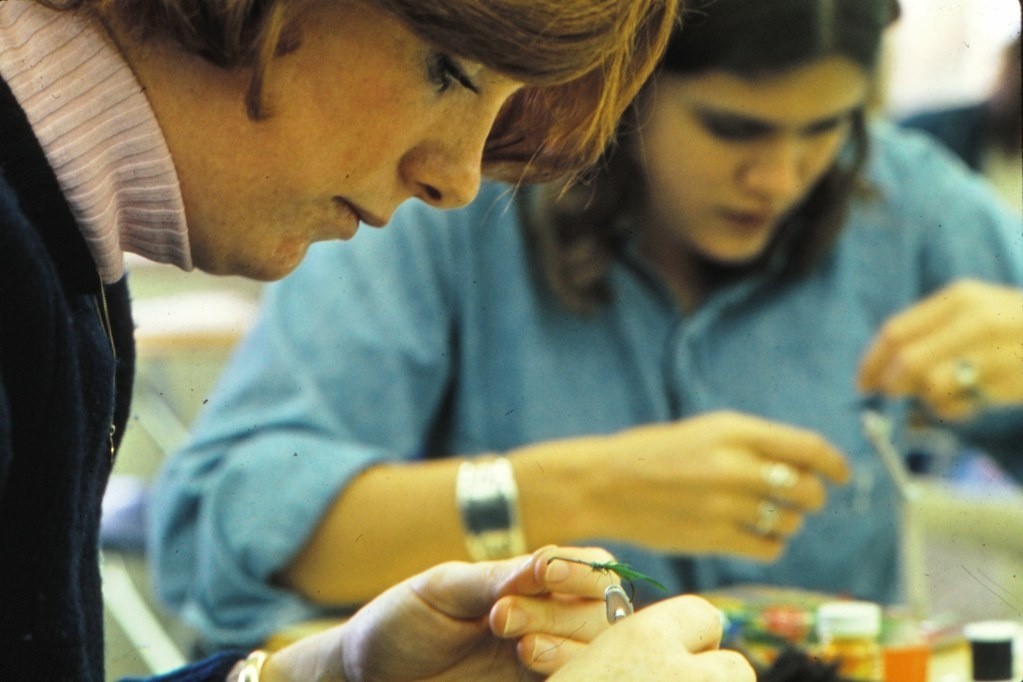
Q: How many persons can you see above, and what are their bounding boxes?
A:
[154,1,1023,652]
[900,28,1023,219]
[0,0,759,681]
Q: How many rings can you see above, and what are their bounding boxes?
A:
[755,497,782,537]
[955,354,982,397]
[764,463,791,500]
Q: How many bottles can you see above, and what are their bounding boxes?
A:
[816,601,885,682]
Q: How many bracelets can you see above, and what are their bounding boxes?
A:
[238,648,269,682]
[457,452,529,564]
